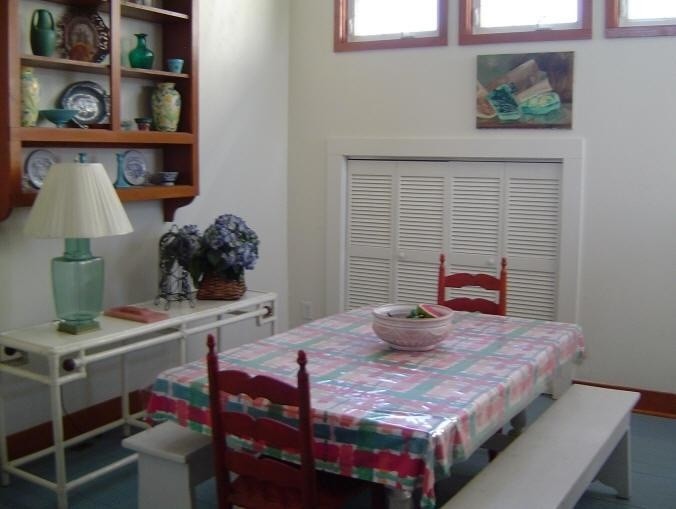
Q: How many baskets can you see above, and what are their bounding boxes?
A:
[197,263,246,300]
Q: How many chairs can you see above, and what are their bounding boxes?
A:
[437,253,507,316]
[207,334,386,509]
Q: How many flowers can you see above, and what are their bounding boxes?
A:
[183,214,259,271]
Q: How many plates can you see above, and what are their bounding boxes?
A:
[24,149,56,189]
[59,79,109,128]
[120,151,148,186]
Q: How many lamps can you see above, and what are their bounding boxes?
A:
[25,163,134,336]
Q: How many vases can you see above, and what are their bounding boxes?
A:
[128,33,156,69]
[151,82,181,132]
[21,65,40,127]
[197,269,246,299]
[30,8,56,57]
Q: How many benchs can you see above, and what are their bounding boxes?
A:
[122,420,216,509]
[439,384,641,509]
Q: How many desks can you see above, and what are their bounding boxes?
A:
[0,288,277,509]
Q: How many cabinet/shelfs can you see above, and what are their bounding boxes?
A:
[0,0,200,219]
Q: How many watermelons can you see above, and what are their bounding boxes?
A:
[418,302,447,318]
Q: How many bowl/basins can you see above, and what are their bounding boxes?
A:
[155,171,180,185]
[371,302,456,352]
[165,58,185,73]
[38,109,79,126]
[134,116,154,132]
